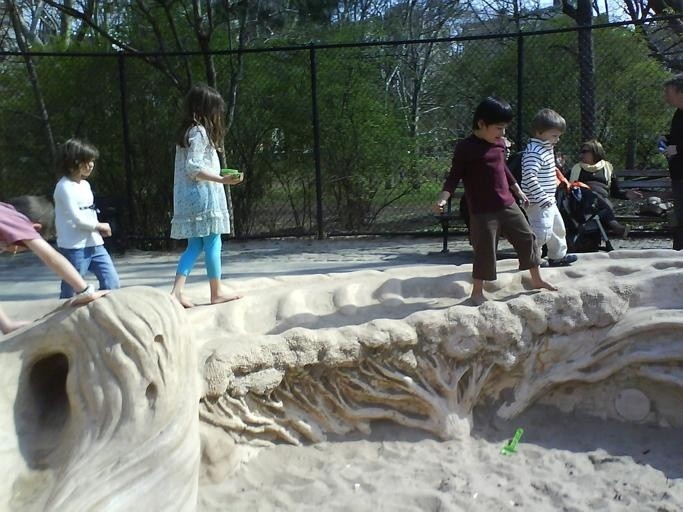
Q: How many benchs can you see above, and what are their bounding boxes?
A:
[431,167,678,255]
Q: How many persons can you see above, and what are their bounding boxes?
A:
[661,78,683,250]
[53,137,121,298]
[170,84,243,308]
[0,195,110,333]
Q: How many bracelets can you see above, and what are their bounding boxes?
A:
[75,284,94,299]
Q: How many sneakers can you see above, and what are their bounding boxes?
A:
[540,254,578,266]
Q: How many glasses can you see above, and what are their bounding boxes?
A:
[580,150,592,153]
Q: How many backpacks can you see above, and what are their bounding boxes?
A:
[507,141,530,201]
